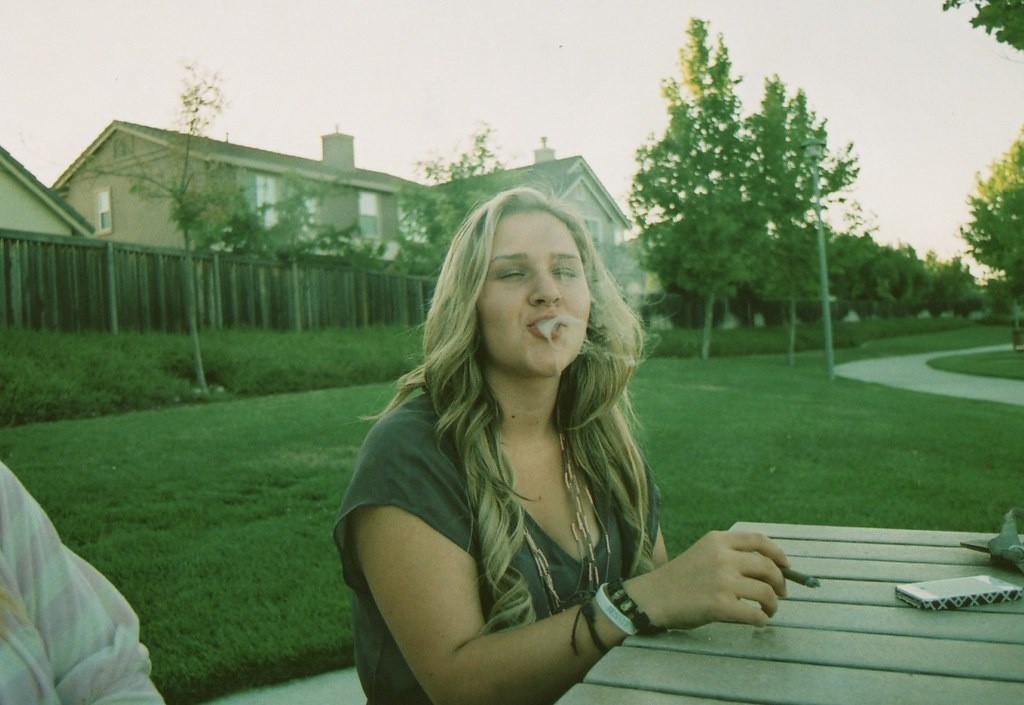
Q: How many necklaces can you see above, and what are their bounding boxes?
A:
[521,432,611,616]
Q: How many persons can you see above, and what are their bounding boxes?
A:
[331,187,790,705]
[0,460,166,705]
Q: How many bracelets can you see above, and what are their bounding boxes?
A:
[570,581,667,654]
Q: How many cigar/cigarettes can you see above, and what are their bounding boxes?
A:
[779,566,822,588]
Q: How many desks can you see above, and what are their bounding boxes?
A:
[552,519,1024,705]
